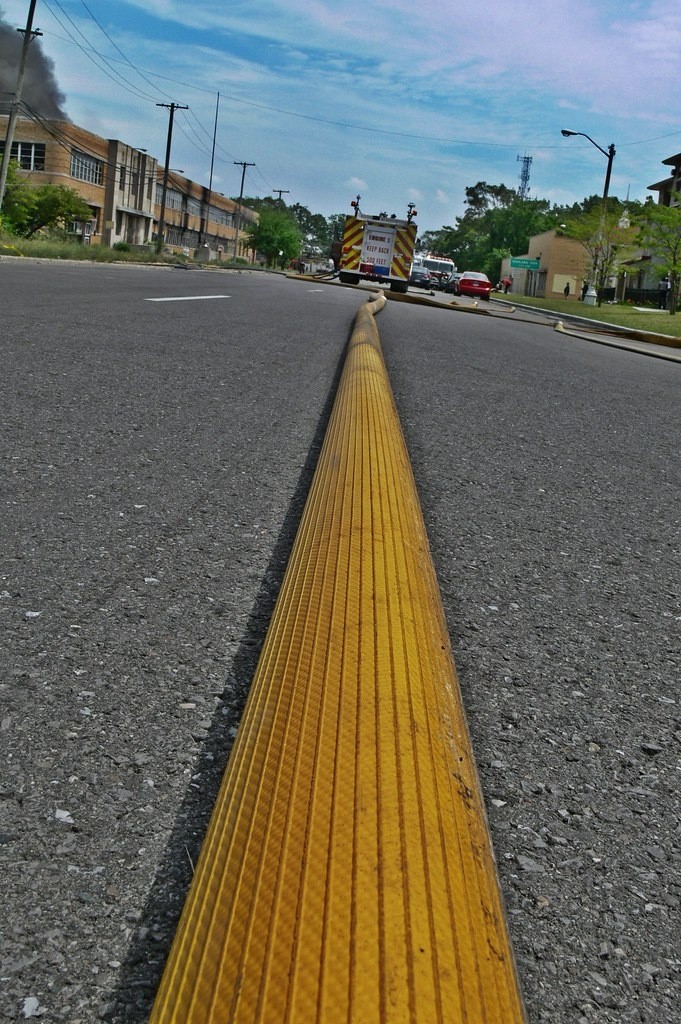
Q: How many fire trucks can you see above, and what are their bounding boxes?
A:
[339,195,419,293]
[423,255,457,289]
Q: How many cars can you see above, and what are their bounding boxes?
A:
[443,272,462,293]
[409,265,431,290]
[430,277,440,290]
[454,271,491,301]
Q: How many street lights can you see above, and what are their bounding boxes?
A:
[123,147,147,243]
[561,128,615,308]
[156,169,184,254]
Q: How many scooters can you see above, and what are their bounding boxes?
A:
[491,281,510,295]
